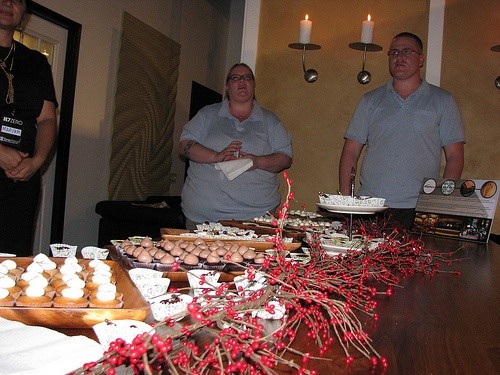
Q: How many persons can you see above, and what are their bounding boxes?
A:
[179,64,293,230]
[339,32,465,227]
[0,1,59,257]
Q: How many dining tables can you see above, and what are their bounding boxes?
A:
[50,239,500,374]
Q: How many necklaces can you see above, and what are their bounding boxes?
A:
[0,39,15,104]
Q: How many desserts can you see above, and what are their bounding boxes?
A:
[0,254,126,308]
[119,209,386,272]
[91,269,267,353]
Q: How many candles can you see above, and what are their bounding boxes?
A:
[360,19,375,44]
[298,20,312,43]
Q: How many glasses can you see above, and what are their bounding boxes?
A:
[387,48,421,56]
[226,73,255,81]
